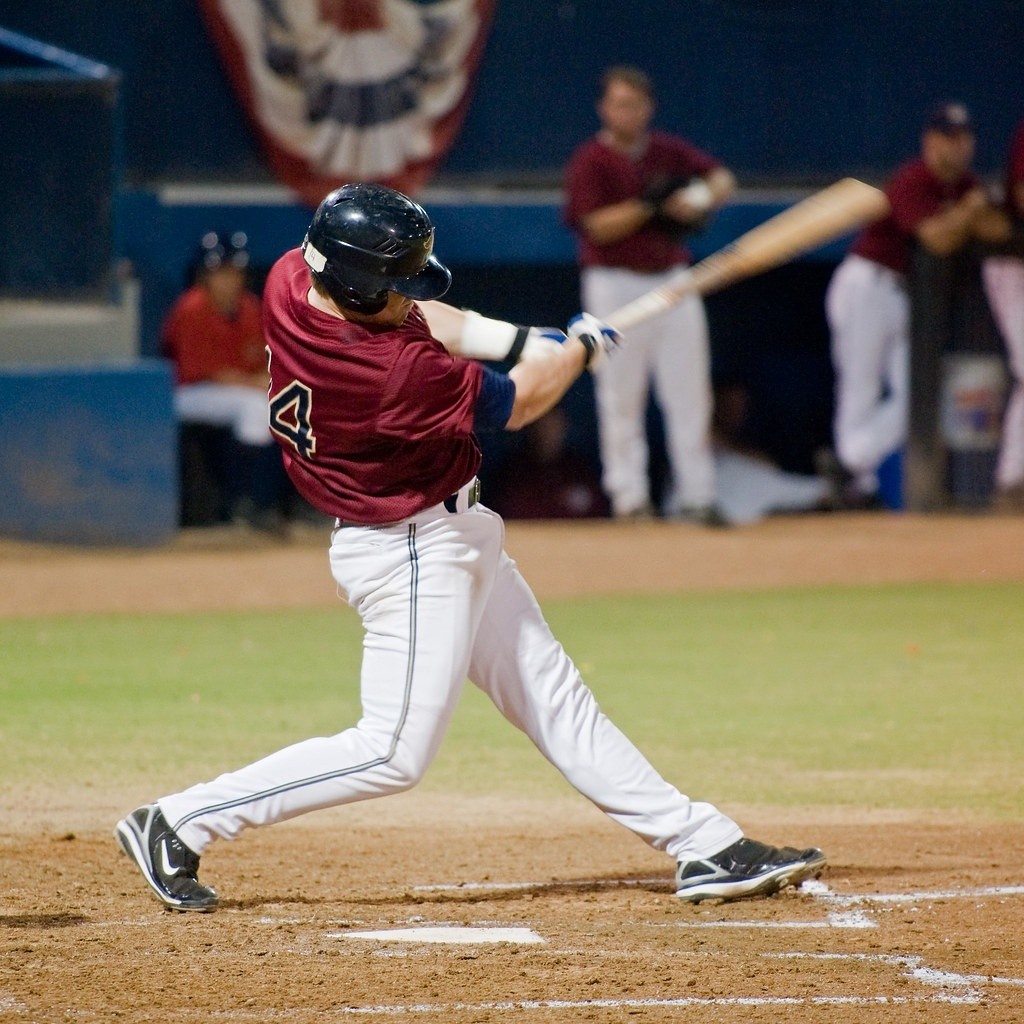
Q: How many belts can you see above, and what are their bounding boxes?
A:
[337,475,483,530]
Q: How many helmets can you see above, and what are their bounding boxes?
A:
[299,180,452,304]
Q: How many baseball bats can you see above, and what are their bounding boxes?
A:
[597,177,893,331]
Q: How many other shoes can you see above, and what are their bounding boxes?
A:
[678,502,730,528]
[627,499,653,517]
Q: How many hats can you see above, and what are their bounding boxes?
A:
[927,105,979,139]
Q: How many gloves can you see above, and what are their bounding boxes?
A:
[565,309,627,374]
[461,307,571,365]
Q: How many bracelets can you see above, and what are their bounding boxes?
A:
[685,178,713,211]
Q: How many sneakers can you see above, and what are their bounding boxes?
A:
[674,838,829,901]
[112,801,221,913]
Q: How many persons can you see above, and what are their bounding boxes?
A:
[827,107,1023,510]
[111,181,824,915]
[558,60,737,524]
[162,239,276,528]
